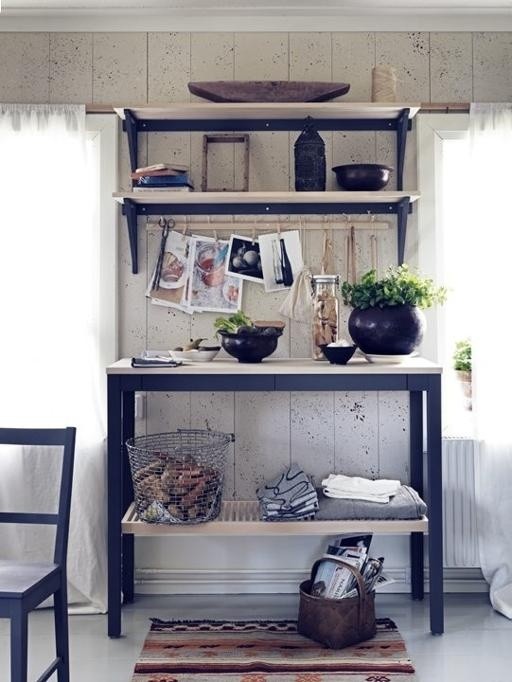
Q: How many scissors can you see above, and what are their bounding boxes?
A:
[152,219,175,291]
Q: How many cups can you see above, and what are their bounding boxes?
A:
[196,241,227,287]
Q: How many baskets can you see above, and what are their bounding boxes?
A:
[127,432,230,526]
[298,559,377,648]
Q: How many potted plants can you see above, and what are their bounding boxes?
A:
[212,311,282,362]
[340,264,449,354]
[454,337,473,411]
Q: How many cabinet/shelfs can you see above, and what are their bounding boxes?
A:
[110,105,421,275]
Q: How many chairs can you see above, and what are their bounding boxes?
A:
[2,427,78,682]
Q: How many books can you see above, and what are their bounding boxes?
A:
[131,161,196,193]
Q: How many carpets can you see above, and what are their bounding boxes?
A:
[131,618,417,681]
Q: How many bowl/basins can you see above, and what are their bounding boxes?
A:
[217,330,283,364]
[318,343,358,366]
[330,162,394,192]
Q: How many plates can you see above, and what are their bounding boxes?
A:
[168,349,220,363]
[153,254,190,289]
[221,279,242,305]
[355,350,421,367]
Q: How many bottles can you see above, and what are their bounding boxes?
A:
[279,237,294,288]
[293,114,328,192]
[309,271,342,361]
[271,240,285,285]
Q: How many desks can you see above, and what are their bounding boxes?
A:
[106,358,444,641]
